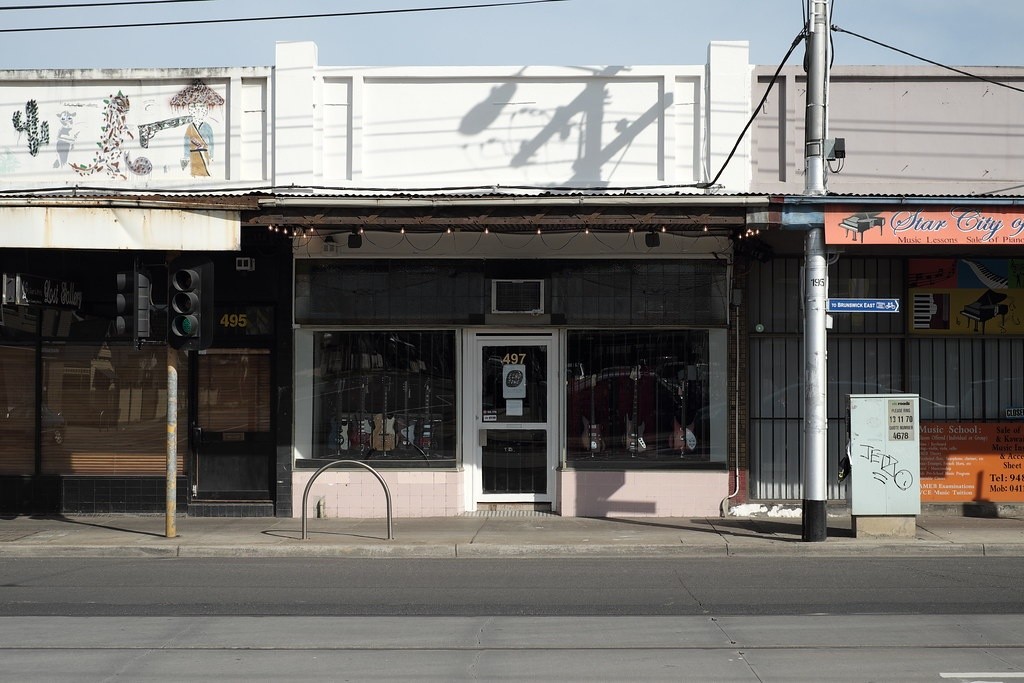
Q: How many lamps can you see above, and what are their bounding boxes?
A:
[347,228,362,249]
[645,226,661,248]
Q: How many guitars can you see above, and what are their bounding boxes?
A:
[326,375,440,456]
[579,364,698,456]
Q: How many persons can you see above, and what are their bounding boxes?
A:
[217,349,249,406]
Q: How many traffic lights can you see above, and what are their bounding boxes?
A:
[166,254,215,351]
[108,259,152,345]
